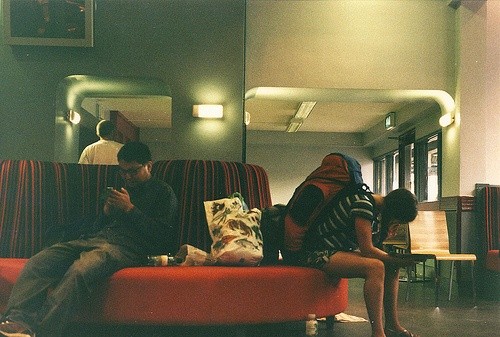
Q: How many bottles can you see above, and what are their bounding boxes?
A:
[305,313,319,337]
[145,254,182,265]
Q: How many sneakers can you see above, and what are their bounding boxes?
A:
[0,319,37,337]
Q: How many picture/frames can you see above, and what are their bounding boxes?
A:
[2,0,93,48]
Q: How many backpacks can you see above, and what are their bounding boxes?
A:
[282,152,377,254]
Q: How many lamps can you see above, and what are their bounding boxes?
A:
[69,109,81,124]
[193,105,223,119]
[438,111,455,128]
[245,112,251,125]
[286,101,317,133]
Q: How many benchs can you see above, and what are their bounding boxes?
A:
[480,185,500,300]
[0,161,348,337]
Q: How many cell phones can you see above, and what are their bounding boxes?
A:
[104,186,113,198]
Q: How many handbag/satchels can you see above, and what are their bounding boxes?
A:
[203,195,264,267]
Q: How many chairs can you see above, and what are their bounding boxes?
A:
[405,211,478,310]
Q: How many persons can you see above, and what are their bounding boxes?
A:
[1,141,179,337]
[301,188,423,337]
[78,120,124,165]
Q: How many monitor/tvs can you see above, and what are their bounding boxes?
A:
[3,0,96,48]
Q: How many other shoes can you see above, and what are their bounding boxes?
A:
[371,326,420,337]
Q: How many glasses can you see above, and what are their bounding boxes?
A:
[119,164,144,176]
